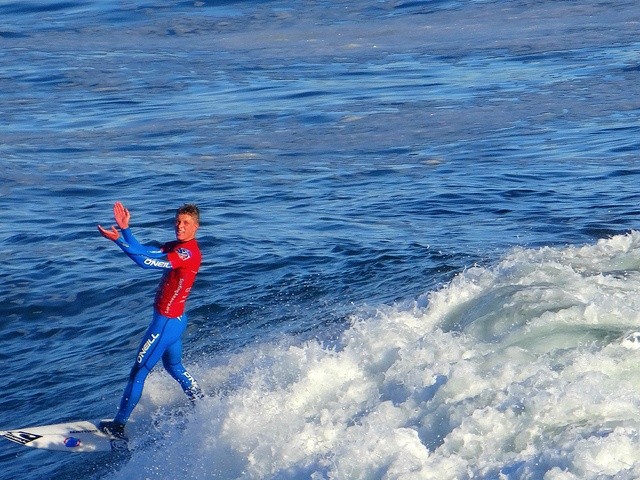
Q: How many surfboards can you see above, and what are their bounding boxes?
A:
[0,419,136,452]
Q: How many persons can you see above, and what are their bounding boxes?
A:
[97,201,205,438]
